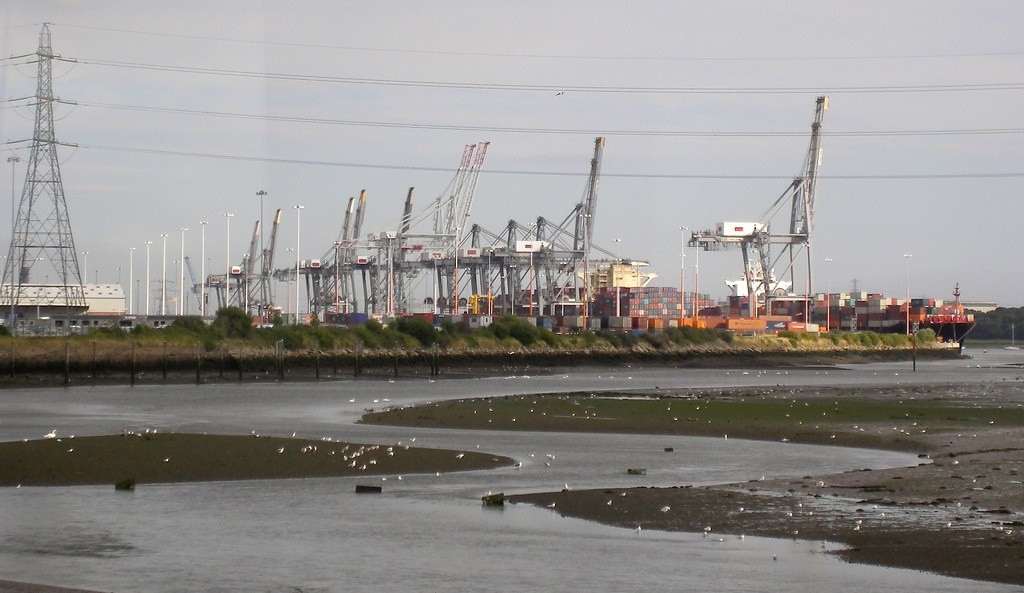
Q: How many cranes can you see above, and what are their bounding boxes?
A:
[176,136,657,333]
[675,93,827,324]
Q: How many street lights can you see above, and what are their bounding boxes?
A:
[452,228,463,315]
[750,249,759,319]
[801,244,812,325]
[432,255,441,314]
[485,250,494,316]
[242,253,250,316]
[143,241,154,317]
[292,206,304,325]
[35,258,44,320]
[526,223,537,315]
[199,220,209,317]
[286,248,294,326]
[82,251,89,285]
[824,258,833,331]
[178,227,190,316]
[255,190,268,329]
[693,235,704,322]
[128,247,136,314]
[385,234,395,315]
[509,264,517,315]
[903,252,913,336]
[678,225,689,318]
[223,212,234,309]
[612,239,623,266]
[332,241,341,316]
[579,214,592,330]
[160,233,169,317]
[386,257,394,312]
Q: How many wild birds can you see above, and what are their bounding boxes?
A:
[555,91,565,97]
[14,361,1024,561]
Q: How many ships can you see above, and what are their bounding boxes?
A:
[421,262,975,356]
